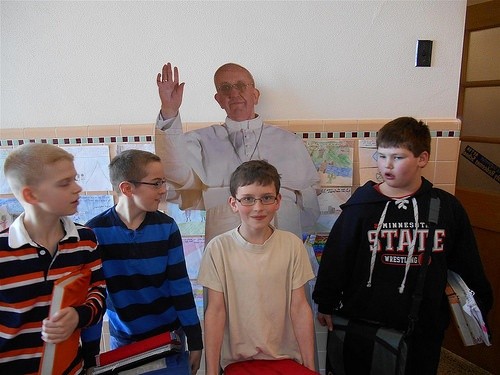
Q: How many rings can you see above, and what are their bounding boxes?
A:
[163,80,167,82]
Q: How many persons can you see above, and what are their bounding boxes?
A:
[312,117,494,375]
[154,62,321,311]
[0,143,107,375]
[83,149,203,375]
[196,160,317,375]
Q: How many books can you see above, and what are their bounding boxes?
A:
[445,270,491,348]
[92,332,183,374]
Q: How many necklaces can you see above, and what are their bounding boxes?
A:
[221,123,265,163]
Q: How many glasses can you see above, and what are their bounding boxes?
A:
[216,82,254,95]
[236,195,277,206]
[128,178,167,188]
